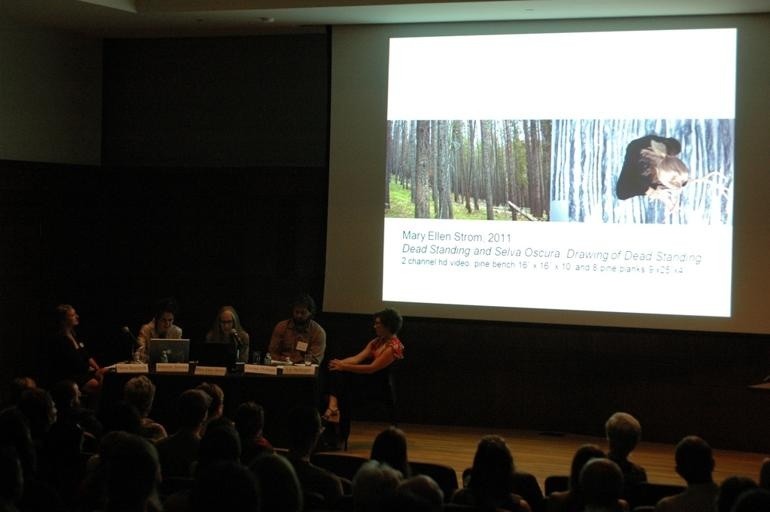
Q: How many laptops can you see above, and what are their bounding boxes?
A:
[150,338,190,364]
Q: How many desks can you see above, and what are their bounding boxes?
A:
[106,361,320,398]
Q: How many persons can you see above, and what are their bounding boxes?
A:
[205,306,250,364]
[0,375,770,512]
[55,304,103,394]
[314,309,404,451]
[268,293,327,365]
[132,297,182,362]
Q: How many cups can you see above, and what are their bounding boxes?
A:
[305,354,313,366]
[253,351,260,364]
[264,356,271,365]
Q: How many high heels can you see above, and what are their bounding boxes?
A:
[322,406,339,423]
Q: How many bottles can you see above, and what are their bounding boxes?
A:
[161,350,168,362]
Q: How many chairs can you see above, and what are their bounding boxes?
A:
[273,454,722,512]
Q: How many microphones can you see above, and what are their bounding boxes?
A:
[122,326,142,350]
[230,329,242,347]
